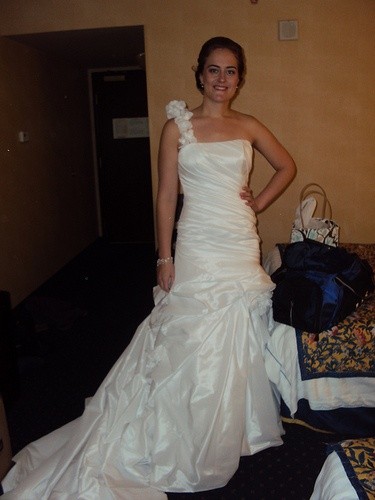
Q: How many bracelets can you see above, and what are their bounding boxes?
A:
[156,257,173,268]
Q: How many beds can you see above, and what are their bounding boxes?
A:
[266,243,375,412]
[310,438,375,500]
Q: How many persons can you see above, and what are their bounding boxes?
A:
[155,36,297,451]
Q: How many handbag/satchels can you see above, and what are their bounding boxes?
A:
[288,182,339,247]
[270,237,373,333]
